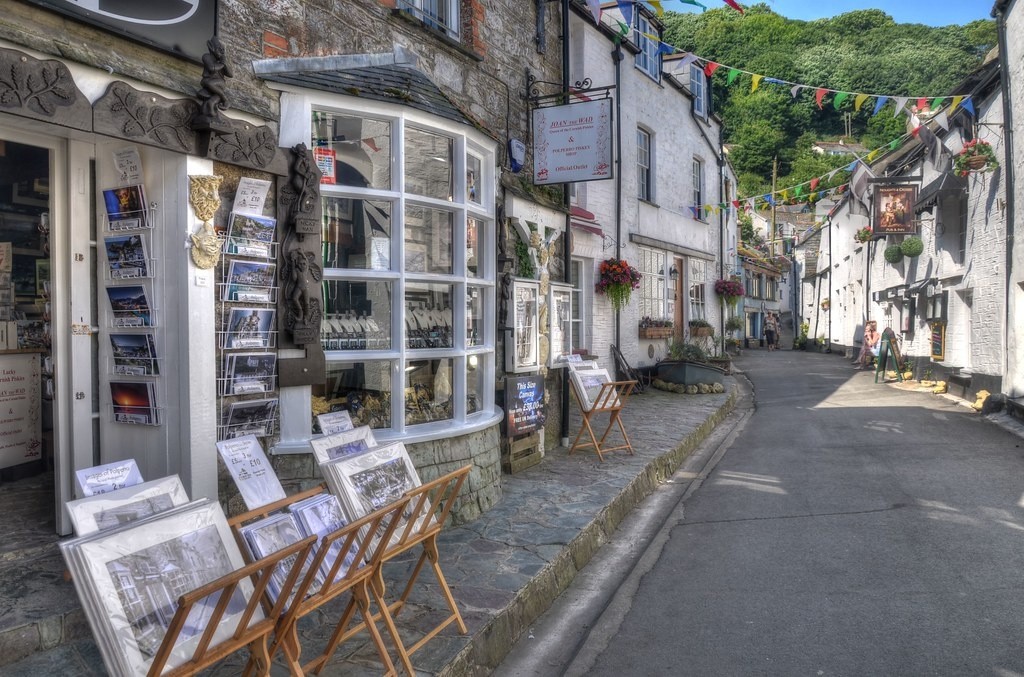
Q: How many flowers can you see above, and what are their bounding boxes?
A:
[595,258,642,310]
[953,138,1000,178]
[714,280,745,309]
[854,226,873,243]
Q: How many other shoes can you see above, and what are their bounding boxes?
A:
[851,361,861,365]
[854,366,864,370]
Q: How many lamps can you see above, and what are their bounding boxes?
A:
[669,263,679,280]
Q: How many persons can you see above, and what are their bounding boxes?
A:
[851,323,881,371]
[762,310,779,352]
[771,312,781,349]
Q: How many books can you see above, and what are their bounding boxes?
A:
[223,259,276,303]
[106,283,155,328]
[109,333,160,377]
[223,176,277,258]
[110,380,161,427]
[224,306,276,348]
[223,398,278,440]
[103,233,150,280]
[223,352,277,395]
[102,183,151,231]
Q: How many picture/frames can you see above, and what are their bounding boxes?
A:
[568,360,620,412]
[57,426,437,677]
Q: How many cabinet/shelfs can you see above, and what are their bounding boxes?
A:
[101,203,164,428]
[216,230,280,442]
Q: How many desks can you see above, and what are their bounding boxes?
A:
[0,348,47,482]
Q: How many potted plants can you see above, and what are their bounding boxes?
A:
[706,333,731,376]
[903,360,915,380]
[920,364,933,387]
[821,299,829,308]
[689,319,714,337]
[639,316,675,339]
[725,316,743,355]
[874,357,878,369]
